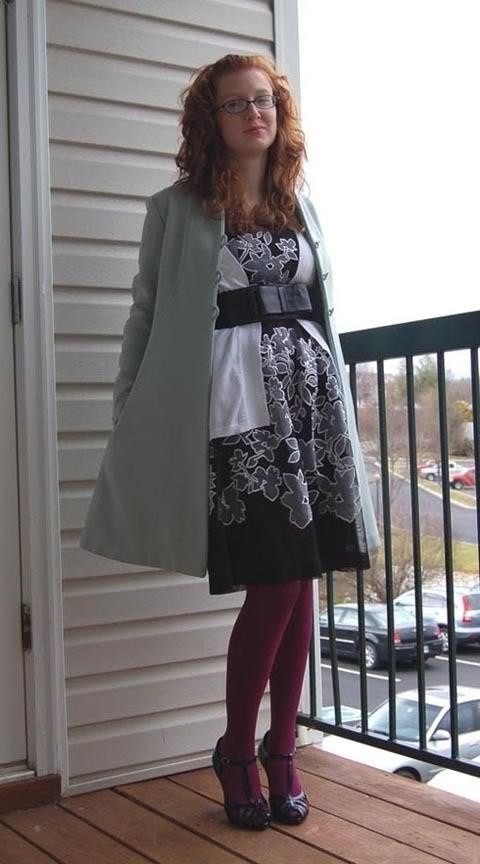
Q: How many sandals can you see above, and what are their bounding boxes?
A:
[212,736,272,830]
[257,730,309,825]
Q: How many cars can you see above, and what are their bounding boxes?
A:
[420,460,467,479]
[417,457,436,472]
[449,467,475,490]
[318,571,480,802]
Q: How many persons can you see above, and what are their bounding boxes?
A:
[81,55,381,828]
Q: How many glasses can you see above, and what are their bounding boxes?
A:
[214,95,282,114]
